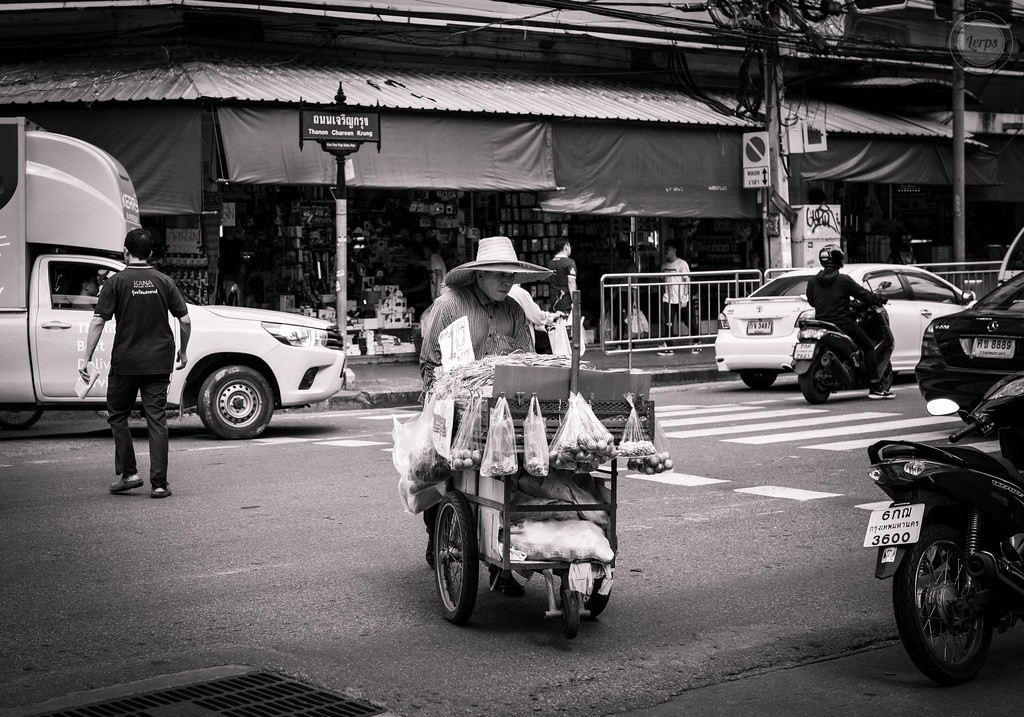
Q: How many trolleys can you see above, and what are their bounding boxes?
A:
[431,396,656,641]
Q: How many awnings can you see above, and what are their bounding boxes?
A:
[0,45,1006,217]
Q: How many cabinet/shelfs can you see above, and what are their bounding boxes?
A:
[286,309,422,362]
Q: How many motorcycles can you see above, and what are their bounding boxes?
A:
[863,369,1024,687]
[790,273,894,405]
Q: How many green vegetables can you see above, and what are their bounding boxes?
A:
[411,449,455,482]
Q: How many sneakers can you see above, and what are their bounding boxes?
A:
[867,389,897,399]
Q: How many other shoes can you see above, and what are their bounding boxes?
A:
[693,346,703,355]
[657,350,675,355]
[490,571,525,597]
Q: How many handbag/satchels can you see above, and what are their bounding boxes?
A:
[547,317,572,355]
[391,387,673,515]
[624,306,650,338]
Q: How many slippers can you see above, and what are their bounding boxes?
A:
[109,474,145,491]
[151,486,170,497]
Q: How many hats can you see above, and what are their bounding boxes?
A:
[445,235,553,287]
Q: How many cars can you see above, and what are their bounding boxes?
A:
[713,263,979,390]
[915,270,1024,423]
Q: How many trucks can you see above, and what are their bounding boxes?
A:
[0,116,350,440]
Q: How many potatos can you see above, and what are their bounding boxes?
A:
[451,448,482,469]
[627,451,674,474]
[548,432,617,470]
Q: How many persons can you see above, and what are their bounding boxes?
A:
[605,241,638,350]
[405,240,449,302]
[78,229,191,496]
[70,270,100,311]
[420,236,554,596]
[548,239,578,337]
[806,244,896,399]
[506,282,565,352]
[657,240,703,355]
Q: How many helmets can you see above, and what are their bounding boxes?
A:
[819,244,843,267]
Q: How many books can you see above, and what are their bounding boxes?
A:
[73,362,101,400]
